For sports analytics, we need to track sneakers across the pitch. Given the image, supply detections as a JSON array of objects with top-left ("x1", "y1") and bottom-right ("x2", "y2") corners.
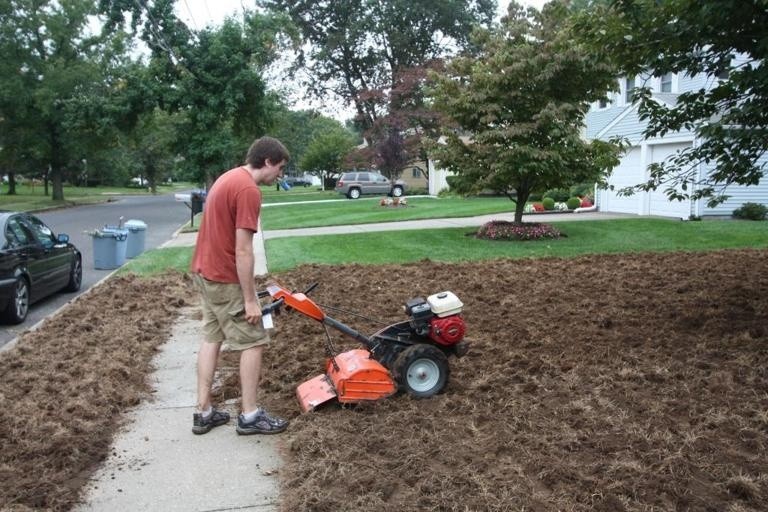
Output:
[
  {"x1": 236, "y1": 408, "x2": 289, "y2": 436},
  {"x1": 192, "y1": 407, "x2": 230, "y2": 435}
]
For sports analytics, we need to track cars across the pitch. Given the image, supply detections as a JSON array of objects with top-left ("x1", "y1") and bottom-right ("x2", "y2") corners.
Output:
[{"x1": 1, "y1": 207, "x2": 84, "y2": 326}]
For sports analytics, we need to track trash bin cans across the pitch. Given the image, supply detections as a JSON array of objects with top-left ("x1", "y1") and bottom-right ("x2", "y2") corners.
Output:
[
  {"x1": 192, "y1": 192, "x2": 205, "y2": 215},
  {"x1": 88, "y1": 219, "x2": 147, "y2": 270}
]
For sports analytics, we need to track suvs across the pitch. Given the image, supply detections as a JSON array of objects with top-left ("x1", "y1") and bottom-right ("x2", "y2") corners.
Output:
[{"x1": 335, "y1": 170, "x2": 411, "y2": 200}]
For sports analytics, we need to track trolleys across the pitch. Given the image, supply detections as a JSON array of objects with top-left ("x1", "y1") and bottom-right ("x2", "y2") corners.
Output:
[{"x1": 245, "y1": 281, "x2": 470, "y2": 416}]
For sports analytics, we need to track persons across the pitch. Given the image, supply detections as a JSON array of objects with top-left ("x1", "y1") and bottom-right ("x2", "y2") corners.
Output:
[{"x1": 189, "y1": 135, "x2": 290, "y2": 434}]
